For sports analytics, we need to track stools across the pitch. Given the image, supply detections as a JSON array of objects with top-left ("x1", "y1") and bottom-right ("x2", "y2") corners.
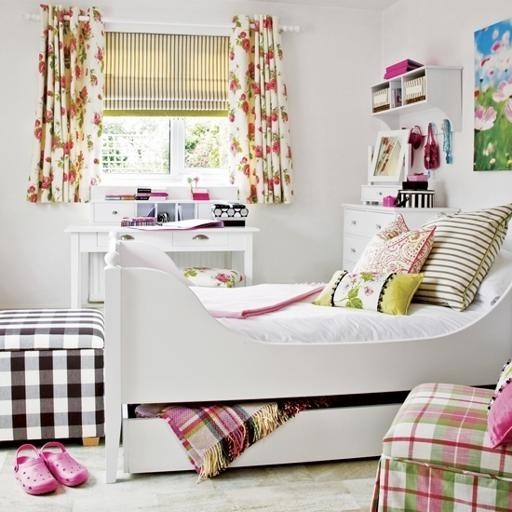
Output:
[
  {"x1": 0, "y1": 307, "x2": 105, "y2": 448},
  {"x1": 179, "y1": 264, "x2": 245, "y2": 290}
]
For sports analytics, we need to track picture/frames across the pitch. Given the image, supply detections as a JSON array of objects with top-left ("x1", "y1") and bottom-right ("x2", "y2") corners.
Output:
[{"x1": 368, "y1": 130, "x2": 412, "y2": 185}]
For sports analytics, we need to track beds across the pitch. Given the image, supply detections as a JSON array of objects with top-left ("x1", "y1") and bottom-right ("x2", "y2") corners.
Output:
[{"x1": 100, "y1": 225, "x2": 511, "y2": 485}]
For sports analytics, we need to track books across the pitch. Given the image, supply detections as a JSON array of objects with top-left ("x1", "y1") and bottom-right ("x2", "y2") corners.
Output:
[{"x1": 119, "y1": 216, "x2": 156, "y2": 225}]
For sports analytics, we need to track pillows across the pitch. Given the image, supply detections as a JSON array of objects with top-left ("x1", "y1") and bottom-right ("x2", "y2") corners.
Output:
[
  {"x1": 416, "y1": 199, "x2": 512, "y2": 309},
  {"x1": 309, "y1": 266, "x2": 423, "y2": 317},
  {"x1": 485, "y1": 356, "x2": 512, "y2": 449},
  {"x1": 475, "y1": 249, "x2": 511, "y2": 305},
  {"x1": 353, "y1": 213, "x2": 440, "y2": 273}
]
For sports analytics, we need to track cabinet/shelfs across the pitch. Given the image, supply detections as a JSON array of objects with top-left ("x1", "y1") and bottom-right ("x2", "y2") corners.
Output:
[
  {"x1": 370, "y1": 66, "x2": 463, "y2": 137},
  {"x1": 360, "y1": 183, "x2": 434, "y2": 206},
  {"x1": 342, "y1": 203, "x2": 456, "y2": 282}
]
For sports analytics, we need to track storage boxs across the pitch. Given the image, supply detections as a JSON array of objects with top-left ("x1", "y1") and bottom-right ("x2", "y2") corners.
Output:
[
  {"x1": 401, "y1": 174, "x2": 429, "y2": 191},
  {"x1": 209, "y1": 202, "x2": 249, "y2": 227}
]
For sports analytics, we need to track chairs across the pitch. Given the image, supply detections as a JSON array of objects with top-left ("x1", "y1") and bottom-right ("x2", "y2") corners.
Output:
[{"x1": 369, "y1": 354, "x2": 512, "y2": 510}]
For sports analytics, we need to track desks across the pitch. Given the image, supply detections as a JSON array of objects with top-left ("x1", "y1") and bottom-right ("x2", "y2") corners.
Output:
[{"x1": 66, "y1": 186, "x2": 261, "y2": 307}]
